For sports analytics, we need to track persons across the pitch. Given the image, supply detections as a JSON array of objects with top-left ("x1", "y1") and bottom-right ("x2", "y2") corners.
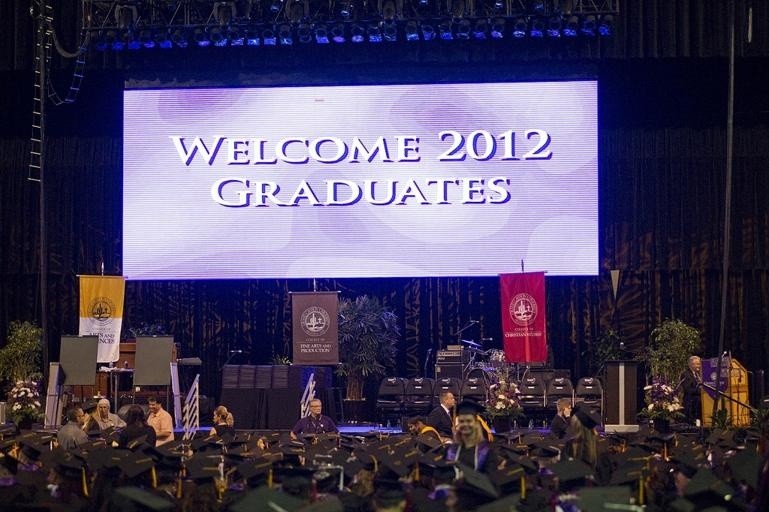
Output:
[{"x1": 0, "y1": 356, "x2": 768, "y2": 512}]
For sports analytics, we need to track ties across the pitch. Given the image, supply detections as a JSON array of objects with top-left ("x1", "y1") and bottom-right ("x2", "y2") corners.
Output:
[{"x1": 694, "y1": 371, "x2": 702, "y2": 385}]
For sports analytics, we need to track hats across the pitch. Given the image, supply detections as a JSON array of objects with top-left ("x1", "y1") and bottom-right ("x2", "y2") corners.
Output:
[{"x1": 0, "y1": 386, "x2": 769, "y2": 511}]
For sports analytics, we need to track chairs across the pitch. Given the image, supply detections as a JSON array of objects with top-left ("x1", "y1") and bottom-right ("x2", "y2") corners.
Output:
[{"x1": 376, "y1": 373, "x2": 604, "y2": 435}]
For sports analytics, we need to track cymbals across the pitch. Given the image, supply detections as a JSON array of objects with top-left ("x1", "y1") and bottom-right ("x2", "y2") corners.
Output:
[
  {"x1": 466, "y1": 347, "x2": 488, "y2": 356},
  {"x1": 461, "y1": 339, "x2": 481, "y2": 347}
]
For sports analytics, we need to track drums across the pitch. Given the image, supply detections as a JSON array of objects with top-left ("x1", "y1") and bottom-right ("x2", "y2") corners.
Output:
[{"x1": 466, "y1": 368, "x2": 490, "y2": 379}]
